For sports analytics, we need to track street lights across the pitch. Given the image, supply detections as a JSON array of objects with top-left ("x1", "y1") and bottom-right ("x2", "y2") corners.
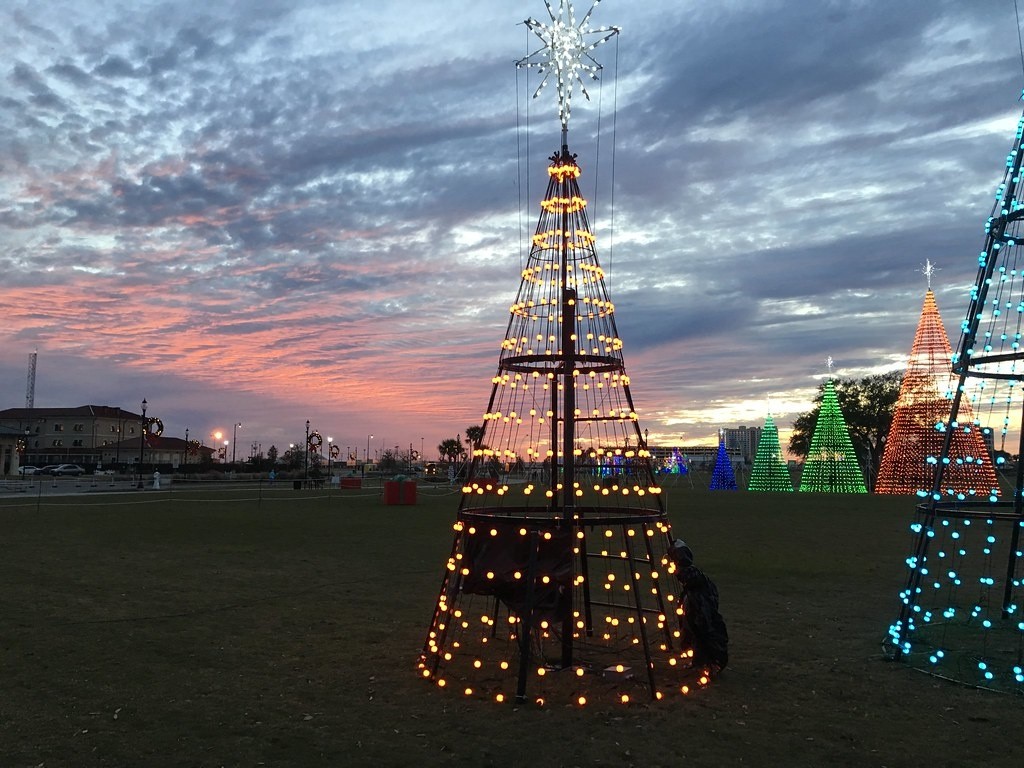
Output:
[
  {"x1": 367, "y1": 433, "x2": 374, "y2": 465},
  {"x1": 452, "y1": 433, "x2": 461, "y2": 478},
  {"x1": 224, "y1": 440, "x2": 229, "y2": 474},
  {"x1": 136, "y1": 397, "x2": 148, "y2": 489},
  {"x1": 326, "y1": 435, "x2": 333, "y2": 480},
  {"x1": 421, "y1": 437, "x2": 424, "y2": 462},
  {"x1": 182, "y1": 428, "x2": 189, "y2": 479},
  {"x1": 231, "y1": 422, "x2": 241, "y2": 474},
  {"x1": 21, "y1": 426, "x2": 31, "y2": 480},
  {"x1": 347, "y1": 446, "x2": 350, "y2": 461},
  {"x1": 303, "y1": 420, "x2": 312, "y2": 487}
]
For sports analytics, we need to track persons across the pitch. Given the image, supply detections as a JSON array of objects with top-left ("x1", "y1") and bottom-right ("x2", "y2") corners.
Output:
[
  {"x1": 152, "y1": 467, "x2": 160, "y2": 489},
  {"x1": 268, "y1": 469, "x2": 277, "y2": 484},
  {"x1": 672, "y1": 538, "x2": 722, "y2": 669}
]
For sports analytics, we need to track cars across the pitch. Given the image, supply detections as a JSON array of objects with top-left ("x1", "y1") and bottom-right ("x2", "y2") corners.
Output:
[
  {"x1": 50, "y1": 464, "x2": 87, "y2": 477},
  {"x1": 33, "y1": 465, "x2": 61, "y2": 475},
  {"x1": 18, "y1": 466, "x2": 39, "y2": 475}
]
